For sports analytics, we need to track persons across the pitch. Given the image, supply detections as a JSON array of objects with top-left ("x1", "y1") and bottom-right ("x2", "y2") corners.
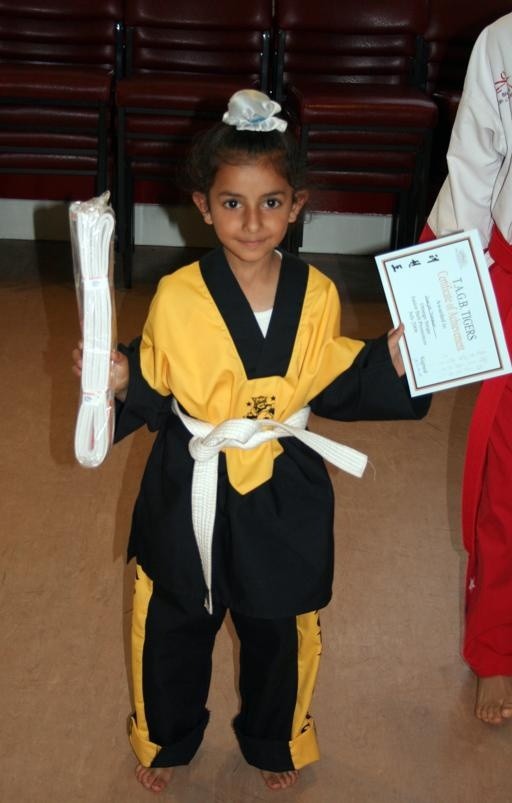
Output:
[
  {"x1": 65, "y1": 86, "x2": 436, "y2": 797},
  {"x1": 412, "y1": 8, "x2": 512, "y2": 731}
]
